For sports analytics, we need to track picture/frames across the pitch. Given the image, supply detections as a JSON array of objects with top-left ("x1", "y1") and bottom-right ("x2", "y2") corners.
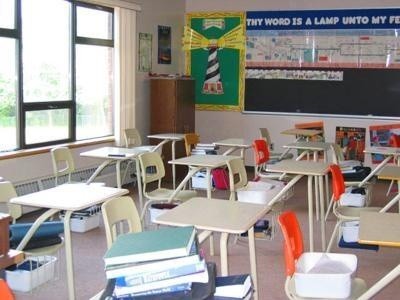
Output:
[{"x1": 185, "y1": 12, "x2": 246, "y2": 112}]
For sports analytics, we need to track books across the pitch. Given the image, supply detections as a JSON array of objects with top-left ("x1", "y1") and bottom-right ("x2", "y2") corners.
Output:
[
  {"x1": 100, "y1": 226, "x2": 256, "y2": 300},
  {"x1": 337, "y1": 156, "x2": 363, "y2": 175},
  {"x1": 62, "y1": 204, "x2": 104, "y2": 219},
  {"x1": 190, "y1": 139, "x2": 219, "y2": 176}
]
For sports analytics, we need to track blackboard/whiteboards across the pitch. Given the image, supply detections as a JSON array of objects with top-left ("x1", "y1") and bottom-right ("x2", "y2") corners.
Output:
[{"x1": 241, "y1": 67, "x2": 400, "y2": 119}]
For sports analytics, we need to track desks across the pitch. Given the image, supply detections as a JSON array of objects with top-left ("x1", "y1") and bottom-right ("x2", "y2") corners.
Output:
[
  {"x1": 0, "y1": 128, "x2": 382, "y2": 300},
  {"x1": 358, "y1": 147, "x2": 400, "y2": 213},
  {"x1": 378, "y1": 166, "x2": 400, "y2": 212},
  {"x1": 356, "y1": 212, "x2": 400, "y2": 300}
]
